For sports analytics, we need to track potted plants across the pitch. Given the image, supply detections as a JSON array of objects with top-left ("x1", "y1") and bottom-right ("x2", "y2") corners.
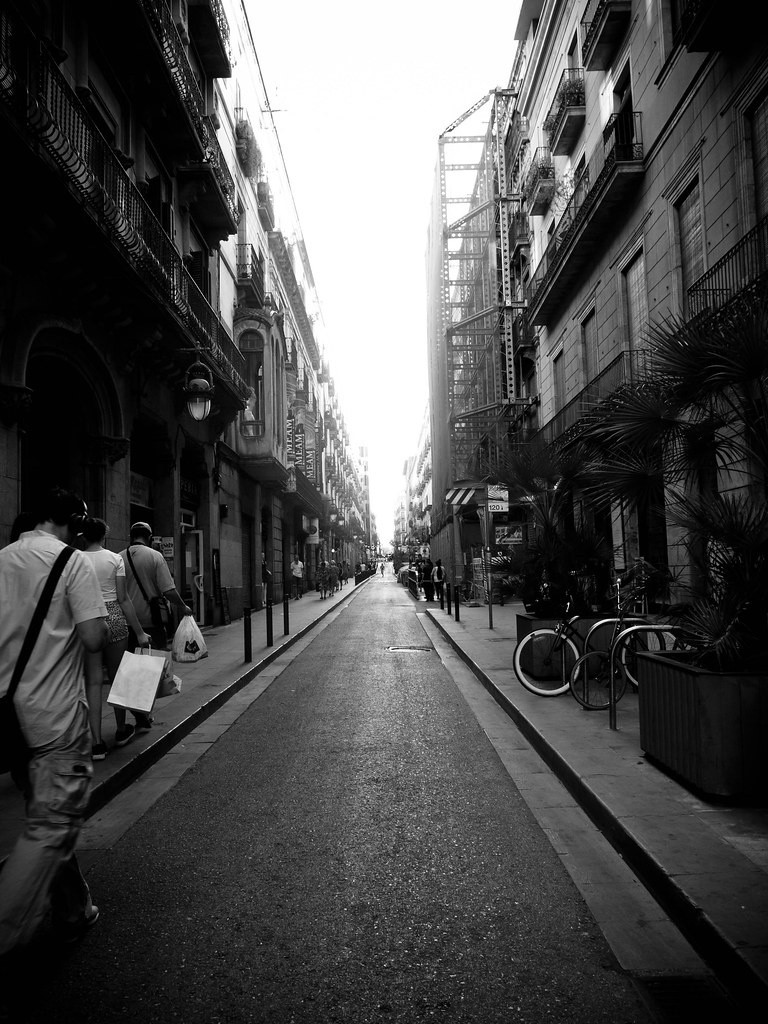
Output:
[
  {"x1": 557, "y1": 261, "x2": 766, "y2": 807},
  {"x1": 477, "y1": 430, "x2": 638, "y2": 679}
]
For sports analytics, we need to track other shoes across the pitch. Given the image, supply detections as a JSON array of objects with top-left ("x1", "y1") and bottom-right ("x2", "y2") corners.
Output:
[
  {"x1": 134, "y1": 720, "x2": 152, "y2": 730},
  {"x1": 58, "y1": 905, "x2": 100, "y2": 936}
]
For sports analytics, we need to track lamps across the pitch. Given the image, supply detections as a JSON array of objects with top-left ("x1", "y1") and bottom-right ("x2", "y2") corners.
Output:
[{"x1": 112, "y1": 343, "x2": 213, "y2": 421}]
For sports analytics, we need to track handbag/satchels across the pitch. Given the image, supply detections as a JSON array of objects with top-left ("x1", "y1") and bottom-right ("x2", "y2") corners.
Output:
[
  {"x1": 0, "y1": 696, "x2": 31, "y2": 774},
  {"x1": 172, "y1": 614, "x2": 209, "y2": 663},
  {"x1": 108, "y1": 641, "x2": 170, "y2": 714},
  {"x1": 134, "y1": 642, "x2": 182, "y2": 699},
  {"x1": 151, "y1": 596, "x2": 171, "y2": 627}
]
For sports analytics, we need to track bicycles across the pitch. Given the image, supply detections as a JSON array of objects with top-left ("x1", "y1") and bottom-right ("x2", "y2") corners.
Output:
[
  {"x1": 569, "y1": 586, "x2": 703, "y2": 711},
  {"x1": 512, "y1": 577, "x2": 671, "y2": 696}
]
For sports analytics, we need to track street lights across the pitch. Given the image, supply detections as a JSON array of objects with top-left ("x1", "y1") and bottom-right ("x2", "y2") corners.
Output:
[{"x1": 168, "y1": 361, "x2": 212, "y2": 630}]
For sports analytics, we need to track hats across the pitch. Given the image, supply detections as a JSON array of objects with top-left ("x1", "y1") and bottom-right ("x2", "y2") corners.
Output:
[{"x1": 130, "y1": 522, "x2": 152, "y2": 536}]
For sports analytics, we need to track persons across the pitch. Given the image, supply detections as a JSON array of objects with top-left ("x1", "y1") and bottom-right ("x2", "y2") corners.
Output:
[
  {"x1": 74, "y1": 517, "x2": 152, "y2": 759},
  {"x1": 0, "y1": 489, "x2": 111, "y2": 960},
  {"x1": 416, "y1": 554, "x2": 445, "y2": 602},
  {"x1": 261, "y1": 552, "x2": 272, "y2": 606},
  {"x1": 290, "y1": 554, "x2": 304, "y2": 600},
  {"x1": 116, "y1": 521, "x2": 193, "y2": 652},
  {"x1": 316, "y1": 558, "x2": 384, "y2": 600}
]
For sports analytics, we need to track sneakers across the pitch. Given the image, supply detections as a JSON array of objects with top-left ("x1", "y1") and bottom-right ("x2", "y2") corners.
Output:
[
  {"x1": 116, "y1": 723, "x2": 135, "y2": 746},
  {"x1": 90, "y1": 738, "x2": 108, "y2": 760}
]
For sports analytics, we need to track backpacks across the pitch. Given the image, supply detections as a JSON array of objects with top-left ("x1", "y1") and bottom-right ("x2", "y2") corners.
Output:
[{"x1": 437, "y1": 567, "x2": 442, "y2": 581}]
